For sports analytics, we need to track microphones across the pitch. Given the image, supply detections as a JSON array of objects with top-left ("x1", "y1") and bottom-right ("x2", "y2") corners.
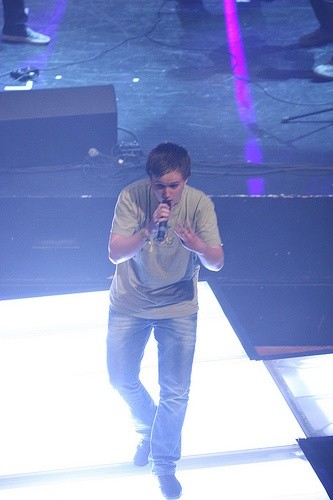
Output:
[{"x1": 157, "y1": 200, "x2": 171, "y2": 241}]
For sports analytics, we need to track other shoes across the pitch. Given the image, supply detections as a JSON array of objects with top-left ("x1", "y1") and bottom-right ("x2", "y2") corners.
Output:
[
  {"x1": 300, "y1": 27, "x2": 333, "y2": 46},
  {"x1": 313, "y1": 65, "x2": 333, "y2": 78}
]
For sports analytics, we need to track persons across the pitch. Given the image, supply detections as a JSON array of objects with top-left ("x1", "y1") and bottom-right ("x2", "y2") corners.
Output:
[
  {"x1": 105, "y1": 143, "x2": 226, "y2": 500},
  {"x1": 0, "y1": 0, "x2": 333, "y2": 80}
]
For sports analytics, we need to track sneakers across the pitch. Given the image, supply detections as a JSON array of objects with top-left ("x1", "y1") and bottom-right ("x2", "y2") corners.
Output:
[
  {"x1": 133, "y1": 430, "x2": 150, "y2": 467},
  {"x1": 157, "y1": 471, "x2": 183, "y2": 500},
  {"x1": 2, "y1": 28, "x2": 50, "y2": 43}
]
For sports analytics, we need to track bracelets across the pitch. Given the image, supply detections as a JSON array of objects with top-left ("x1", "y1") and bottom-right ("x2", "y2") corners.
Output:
[{"x1": 140, "y1": 230, "x2": 148, "y2": 243}]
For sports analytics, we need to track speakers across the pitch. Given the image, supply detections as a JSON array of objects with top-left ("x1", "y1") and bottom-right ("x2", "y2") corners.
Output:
[{"x1": 0, "y1": 84, "x2": 117, "y2": 173}]
